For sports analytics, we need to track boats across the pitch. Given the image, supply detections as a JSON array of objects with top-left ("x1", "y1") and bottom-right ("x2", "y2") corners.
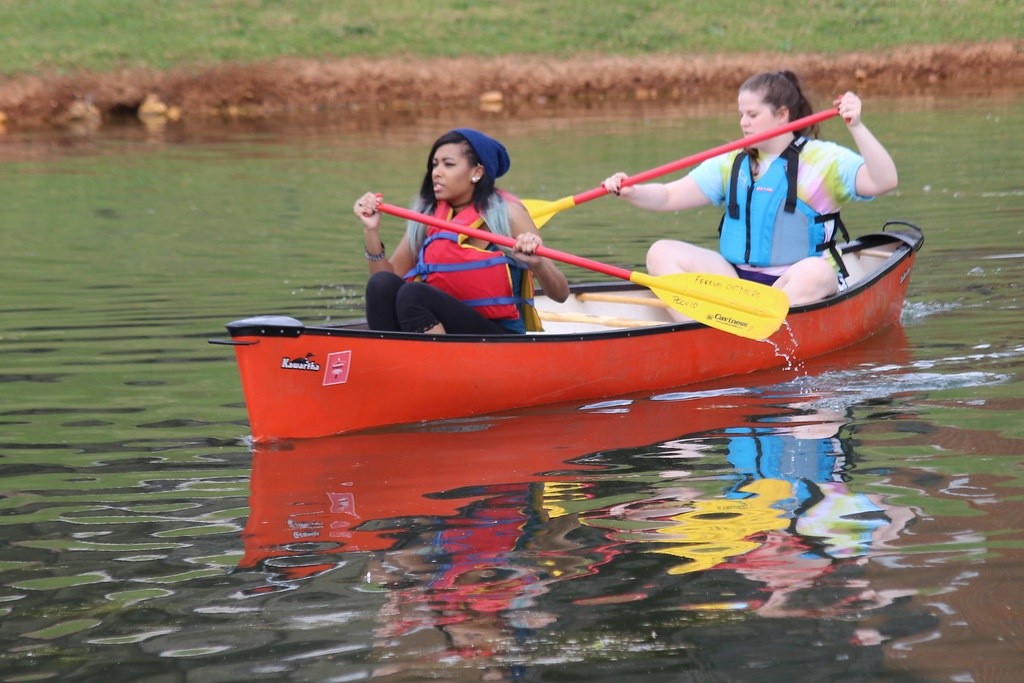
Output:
[
  {"x1": 208, "y1": 222, "x2": 924, "y2": 441},
  {"x1": 230, "y1": 323, "x2": 916, "y2": 576}
]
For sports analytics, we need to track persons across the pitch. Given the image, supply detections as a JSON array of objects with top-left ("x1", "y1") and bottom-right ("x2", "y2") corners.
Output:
[
  {"x1": 354, "y1": 130, "x2": 570, "y2": 335},
  {"x1": 600, "y1": 70, "x2": 897, "y2": 323}
]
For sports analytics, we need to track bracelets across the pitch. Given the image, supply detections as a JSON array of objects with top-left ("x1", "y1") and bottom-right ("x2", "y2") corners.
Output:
[{"x1": 364, "y1": 241, "x2": 385, "y2": 261}]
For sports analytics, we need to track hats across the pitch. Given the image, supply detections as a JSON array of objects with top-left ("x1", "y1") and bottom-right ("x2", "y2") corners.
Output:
[{"x1": 450, "y1": 128, "x2": 510, "y2": 184}]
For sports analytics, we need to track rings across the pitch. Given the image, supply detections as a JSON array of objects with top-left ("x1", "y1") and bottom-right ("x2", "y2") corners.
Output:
[
  {"x1": 359, "y1": 202, "x2": 363, "y2": 206},
  {"x1": 529, "y1": 237, "x2": 534, "y2": 240}
]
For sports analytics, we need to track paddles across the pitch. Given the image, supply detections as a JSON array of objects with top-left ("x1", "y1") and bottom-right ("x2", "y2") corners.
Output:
[
  {"x1": 519, "y1": 93, "x2": 854, "y2": 230},
  {"x1": 362, "y1": 190, "x2": 792, "y2": 341}
]
